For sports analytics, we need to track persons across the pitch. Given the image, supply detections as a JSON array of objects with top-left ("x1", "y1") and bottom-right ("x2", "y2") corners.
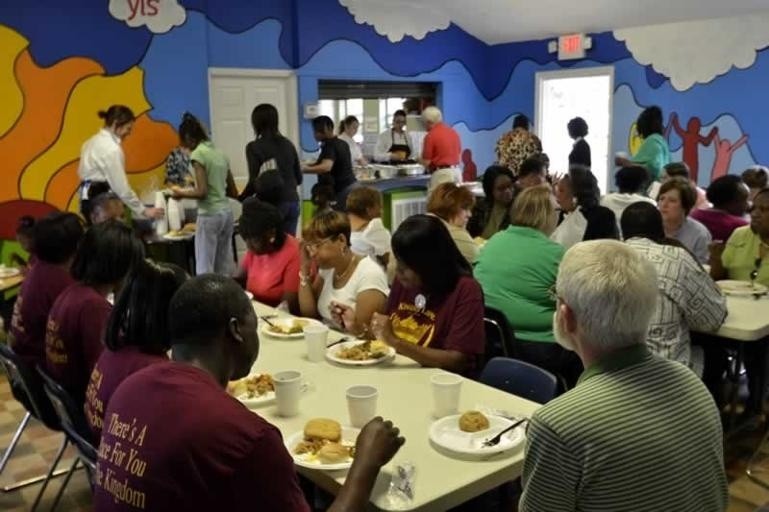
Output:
[
  {"x1": 74, "y1": 105, "x2": 166, "y2": 228},
  {"x1": 231, "y1": 198, "x2": 316, "y2": 319},
  {"x1": 297, "y1": 209, "x2": 390, "y2": 337},
  {"x1": 166, "y1": 124, "x2": 213, "y2": 201},
  {"x1": 494, "y1": 114, "x2": 543, "y2": 177},
  {"x1": 335, "y1": 114, "x2": 367, "y2": 167},
  {"x1": 705, "y1": 185, "x2": 769, "y2": 288},
  {"x1": 330, "y1": 215, "x2": 486, "y2": 373},
  {"x1": 425, "y1": 181, "x2": 480, "y2": 271},
  {"x1": 742, "y1": 164, "x2": 769, "y2": 195},
  {"x1": 418, "y1": 107, "x2": 464, "y2": 170},
  {"x1": 166, "y1": 112, "x2": 239, "y2": 280},
  {"x1": 9, "y1": 213, "x2": 87, "y2": 374},
  {"x1": 97, "y1": 272, "x2": 407, "y2": 511},
  {"x1": 7, "y1": 216, "x2": 38, "y2": 268},
  {"x1": 516, "y1": 156, "x2": 547, "y2": 188},
  {"x1": 599, "y1": 167, "x2": 660, "y2": 235},
  {"x1": 518, "y1": 238, "x2": 733, "y2": 511},
  {"x1": 704, "y1": 174, "x2": 751, "y2": 218},
  {"x1": 474, "y1": 183, "x2": 585, "y2": 385},
  {"x1": 533, "y1": 152, "x2": 552, "y2": 183},
  {"x1": 46, "y1": 218, "x2": 146, "y2": 413},
  {"x1": 86, "y1": 261, "x2": 190, "y2": 439},
  {"x1": 618, "y1": 201, "x2": 727, "y2": 368},
  {"x1": 87, "y1": 193, "x2": 127, "y2": 228},
  {"x1": 467, "y1": 164, "x2": 524, "y2": 241},
  {"x1": 565, "y1": 117, "x2": 592, "y2": 173},
  {"x1": 305, "y1": 115, "x2": 358, "y2": 204},
  {"x1": 552, "y1": 166, "x2": 622, "y2": 242},
  {"x1": 239, "y1": 104, "x2": 304, "y2": 239},
  {"x1": 373, "y1": 109, "x2": 418, "y2": 164},
  {"x1": 663, "y1": 162, "x2": 712, "y2": 213},
  {"x1": 614, "y1": 104, "x2": 670, "y2": 180},
  {"x1": 655, "y1": 177, "x2": 713, "y2": 267}
]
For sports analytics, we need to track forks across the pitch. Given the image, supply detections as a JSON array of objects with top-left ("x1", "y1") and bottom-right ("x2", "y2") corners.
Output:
[{"x1": 482, "y1": 418, "x2": 528, "y2": 447}]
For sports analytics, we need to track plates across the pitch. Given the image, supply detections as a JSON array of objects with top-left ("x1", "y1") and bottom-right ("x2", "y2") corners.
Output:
[
  {"x1": 282, "y1": 425, "x2": 364, "y2": 470},
  {"x1": 428, "y1": 413, "x2": 527, "y2": 456},
  {"x1": 163, "y1": 233, "x2": 193, "y2": 241},
  {"x1": 263, "y1": 317, "x2": 328, "y2": 338},
  {"x1": 327, "y1": 340, "x2": 396, "y2": 365},
  {"x1": 0, "y1": 267, "x2": 21, "y2": 278},
  {"x1": 715, "y1": 280, "x2": 767, "y2": 296},
  {"x1": 225, "y1": 373, "x2": 276, "y2": 405}
]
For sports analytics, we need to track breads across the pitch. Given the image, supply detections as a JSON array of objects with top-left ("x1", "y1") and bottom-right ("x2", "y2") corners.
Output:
[
  {"x1": 305, "y1": 418, "x2": 342, "y2": 445},
  {"x1": 459, "y1": 411, "x2": 488, "y2": 432},
  {"x1": 318, "y1": 444, "x2": 347, "y2": 465}
]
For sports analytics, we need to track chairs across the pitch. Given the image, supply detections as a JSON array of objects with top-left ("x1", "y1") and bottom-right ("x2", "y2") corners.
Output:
[
  {"x1": 34, "y1": 365, "x2": 81, "y2": 512},
  {"x1": 51, "y1": 420, "x2": 98, "y2": 512},
  {"x1": 0, "y1": 344, "x2": 67, "y2": 512},
  {"x1": 480, "y1": 357, "x2": 556, "y2": 405},
  {"x1": 482, "y1": 305, "x2": 515, "y2": 357}
]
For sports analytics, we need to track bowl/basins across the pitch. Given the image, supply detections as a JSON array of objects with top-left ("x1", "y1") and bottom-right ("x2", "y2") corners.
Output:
[
  {"x1": 399, "y1": 163, "x2": 426, "y2": 177},
  {"x1": 372, "y1": 164, "x2": 398, "y2": 180},
  {"x1": 353, "y1": 166, "x2": 373, "y2": 181}
]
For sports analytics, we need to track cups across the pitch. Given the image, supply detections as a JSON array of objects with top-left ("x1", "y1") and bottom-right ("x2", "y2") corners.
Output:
[
  {"x1": 153, "y1": 192, "x2": 168, "y2": 236},
  {"x1": 168, "y1": 196, "x2": 187, "y2": 231},
  {"x1": 429, "y1": 373, "x2": 463, "y2": 418},
  {"x1": 273, "y1": 369, "x2": 305, "y2": 417},
  {"x1": 346, "y1": 385, "x2": 380, "y2": 427},
  {"x1": 303, "y1": 325, "x2": 329, "y2": 361}
]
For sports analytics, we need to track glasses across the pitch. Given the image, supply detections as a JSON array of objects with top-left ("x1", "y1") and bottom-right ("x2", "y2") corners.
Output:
[
  {"x1": 495, "y1": 182, "x2": 513, "y2": 191},
  {"x1": 548, "y1": 286, "x2": 565, "y2": 306},
  {"x1": 750, "y1": 257, "x2": 761, "y2": 280},
  {"x1": 304, "y1": 235, "x2": 335, "y2": 251}
]
